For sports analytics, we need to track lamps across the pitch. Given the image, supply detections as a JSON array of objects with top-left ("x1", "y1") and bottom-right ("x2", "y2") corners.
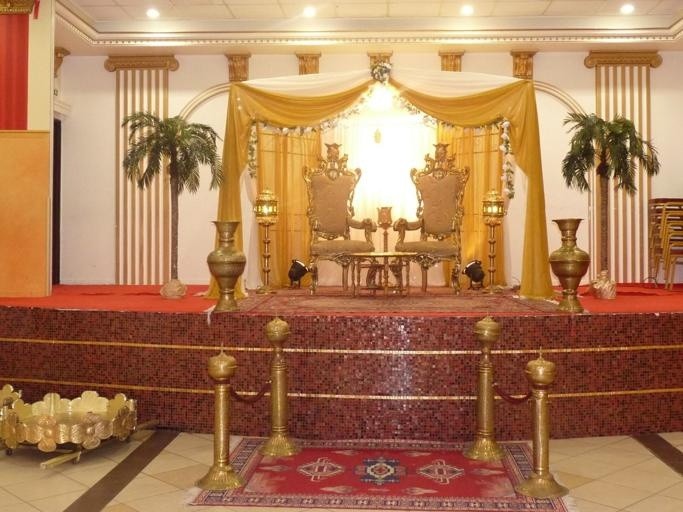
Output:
[
  {"x1": 481, "y1": 187, "x2": 505, "y2": 293},
  {"x1": 252, "y1": 182, "x2": 278, "y2": 295}
]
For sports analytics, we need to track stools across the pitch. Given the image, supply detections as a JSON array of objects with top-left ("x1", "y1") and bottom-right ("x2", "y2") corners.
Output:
[{"x1": 645, "y1": 198, "x2": 683, "y2": 290}]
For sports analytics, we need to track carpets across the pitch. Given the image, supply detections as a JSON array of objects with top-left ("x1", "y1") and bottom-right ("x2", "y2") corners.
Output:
[
  {"x1": 202, "y1": 287, "x2": 589, "y2": 315},
  {"x1": 181, "y1": 436, "x2": 576, "y2": 511}
]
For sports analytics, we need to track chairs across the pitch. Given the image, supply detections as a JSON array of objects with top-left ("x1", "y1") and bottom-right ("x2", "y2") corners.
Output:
[{"x1": 301, "y1": 142, "x2": 470, "y2": 298}]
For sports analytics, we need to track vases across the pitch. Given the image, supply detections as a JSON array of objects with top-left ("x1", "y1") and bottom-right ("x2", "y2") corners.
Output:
[
  {"x1": 548, "y1": 218, "x2": 590, "y2": 313},
  {"x1": 206, "y1": 220, "x2": 245, "y2": 312}
]
